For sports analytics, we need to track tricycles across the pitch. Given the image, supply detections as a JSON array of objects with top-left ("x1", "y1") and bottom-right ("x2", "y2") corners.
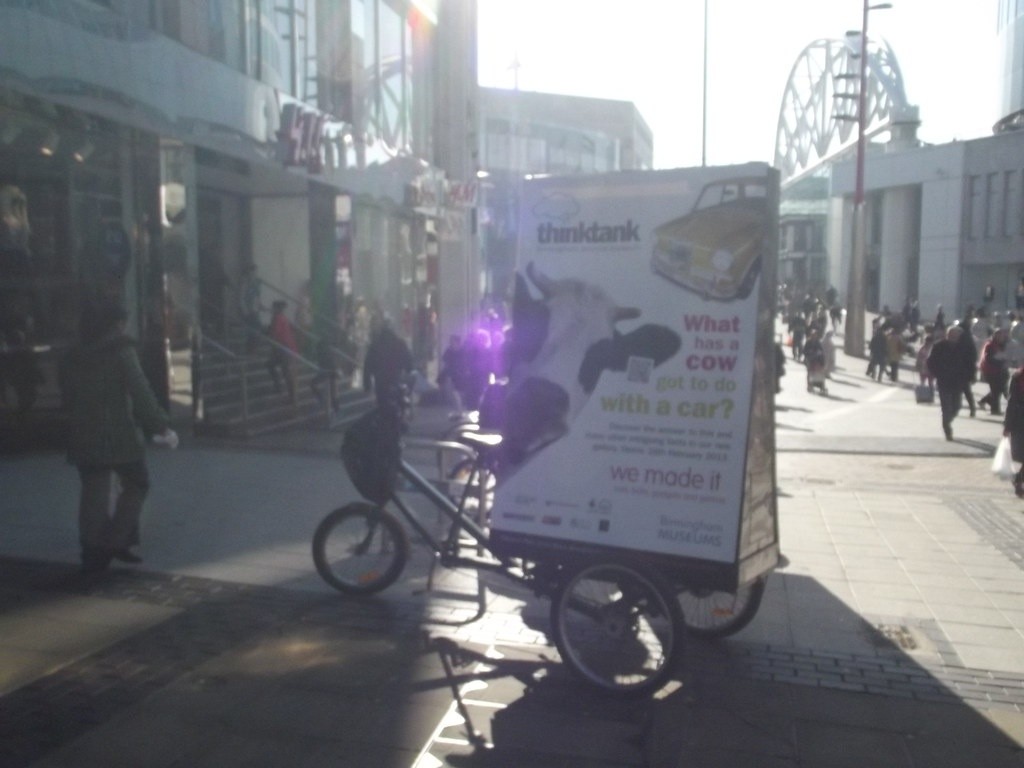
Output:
[{"x1": 307, "y1": 353, "x2": 790, "y2": 696}]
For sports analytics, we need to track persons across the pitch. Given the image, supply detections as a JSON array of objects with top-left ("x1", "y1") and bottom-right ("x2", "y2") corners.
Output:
[
  {"x1": 346, "y1": 301, "x2": 511, "y2": 428},
  {"x1": 774, "y1": 274, "x2": 1024, "y2": 501},
  {"x1": 0, "y1": 150, "x2": 136, "y2": 411},
  {"x1": 265, "y1": 297, "x2": 301, "y2": 405},
  {"x1": 242, "y1": 278, "x2": 270, "y2": 351},
  {"x1": 310, "y1": 332, "x2": 341, "y2": 416},
  {"x1": 64, "y1": 298, "x2": 177, "y2": 572}
]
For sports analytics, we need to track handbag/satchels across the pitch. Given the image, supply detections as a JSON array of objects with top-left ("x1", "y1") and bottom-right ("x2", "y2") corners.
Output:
[
  {"x1": 992, "y1": 436, "x2": 1016, "y2": 481},
  {"x1": 912, "y1": 365, "x2": 933, "y2": 403}
]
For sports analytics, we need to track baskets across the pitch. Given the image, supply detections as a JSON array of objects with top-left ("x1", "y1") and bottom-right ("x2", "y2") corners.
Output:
[{"x1": 342, "y1": 412, "x2": 399, "y2": 503}]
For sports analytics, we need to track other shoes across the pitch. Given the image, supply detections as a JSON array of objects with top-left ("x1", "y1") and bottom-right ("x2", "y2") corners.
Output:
[{"x1": 101, "y1": 540, "x2": 143, "y2": 564}]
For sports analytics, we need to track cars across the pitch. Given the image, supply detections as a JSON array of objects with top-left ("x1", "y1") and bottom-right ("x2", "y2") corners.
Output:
[{"x1": 650, "y1": 169, "x2": 771, "y2": 303}]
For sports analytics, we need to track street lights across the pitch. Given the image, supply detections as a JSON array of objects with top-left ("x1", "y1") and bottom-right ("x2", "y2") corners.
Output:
[{"x1": 831, "y1": 0, "x2": 894, "y2": 356}]
